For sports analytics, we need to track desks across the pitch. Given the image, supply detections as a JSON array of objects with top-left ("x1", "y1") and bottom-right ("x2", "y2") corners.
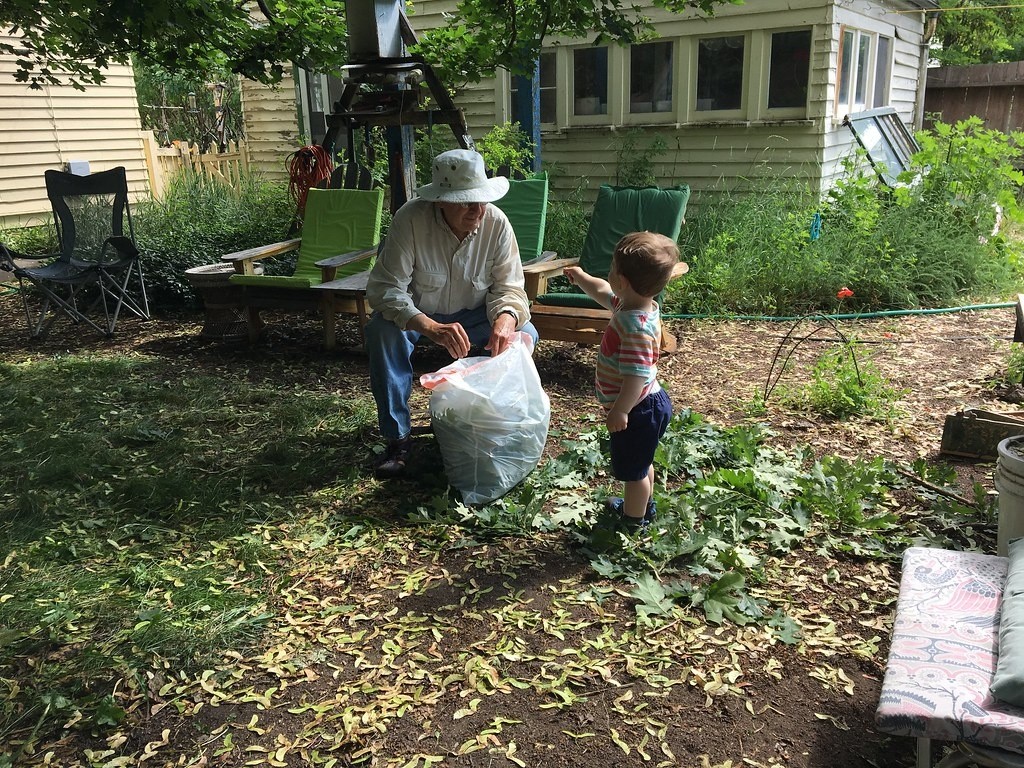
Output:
[{"x1": 310, "y1": 270, "x2": 371, "y2": 344}]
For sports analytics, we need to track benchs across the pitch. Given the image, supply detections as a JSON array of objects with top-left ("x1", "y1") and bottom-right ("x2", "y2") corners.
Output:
[{"x1": 876, "y1": 538, "x2": 1024, "y2": 768}]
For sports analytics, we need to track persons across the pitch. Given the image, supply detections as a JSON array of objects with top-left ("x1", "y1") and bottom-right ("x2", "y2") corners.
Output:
[
  {"x1": 562, "y1": 228, "x2": 680, "y2": 540},
  {"x1": 366, "y1": 148, "x2": 540, "y2": 479}
]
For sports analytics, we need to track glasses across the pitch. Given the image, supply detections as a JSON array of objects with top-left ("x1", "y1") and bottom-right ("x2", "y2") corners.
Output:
[{"x1": 459, "y1": 203, "x2": 472, "y2": 208}]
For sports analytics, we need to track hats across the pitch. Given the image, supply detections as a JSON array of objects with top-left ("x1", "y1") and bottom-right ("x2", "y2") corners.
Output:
[{"x1": 413, "y1": 149, "x2": 512, "y2": 204}]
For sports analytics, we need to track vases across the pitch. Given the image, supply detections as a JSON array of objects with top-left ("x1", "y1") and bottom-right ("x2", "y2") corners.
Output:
[
  {"x1": 996, "y1": 436, "x2": 1024, "y2": 555},
  {"x1": 185, "y1": 263, "x2": 265, "y2": 308}
]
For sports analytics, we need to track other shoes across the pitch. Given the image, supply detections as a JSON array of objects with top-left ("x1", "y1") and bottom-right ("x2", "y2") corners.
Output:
[{"x1": 375, "y1": 434, "x2": 410, "y2": 477}]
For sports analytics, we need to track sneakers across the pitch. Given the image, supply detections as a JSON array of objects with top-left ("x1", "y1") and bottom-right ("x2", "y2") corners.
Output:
[
  {"x1": 599, "y1": 514, "x2": 650, "y2": 537},
  {"x1": 606, "y1": 495, "x2": 659, "y2": 522}
]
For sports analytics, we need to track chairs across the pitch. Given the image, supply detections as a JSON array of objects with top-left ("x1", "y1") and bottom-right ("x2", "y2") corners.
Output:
[
  {"x1": 490, "y1": 179, "x2": 557, "y2": 268},
  {"x1": 522, "y1": 183, "x2": 690, "y2": 353},
  {"x1": 1, "y1": 167, "x2": 151, "y2": 342},
  {"x1": 221, "y1": 186, "x2": 385, "y2": 351}
]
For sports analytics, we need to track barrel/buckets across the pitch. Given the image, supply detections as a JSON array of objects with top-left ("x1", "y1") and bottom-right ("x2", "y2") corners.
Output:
[{"x1": 994, "y1": 435, "x2": 1024, "y2": 557}]
